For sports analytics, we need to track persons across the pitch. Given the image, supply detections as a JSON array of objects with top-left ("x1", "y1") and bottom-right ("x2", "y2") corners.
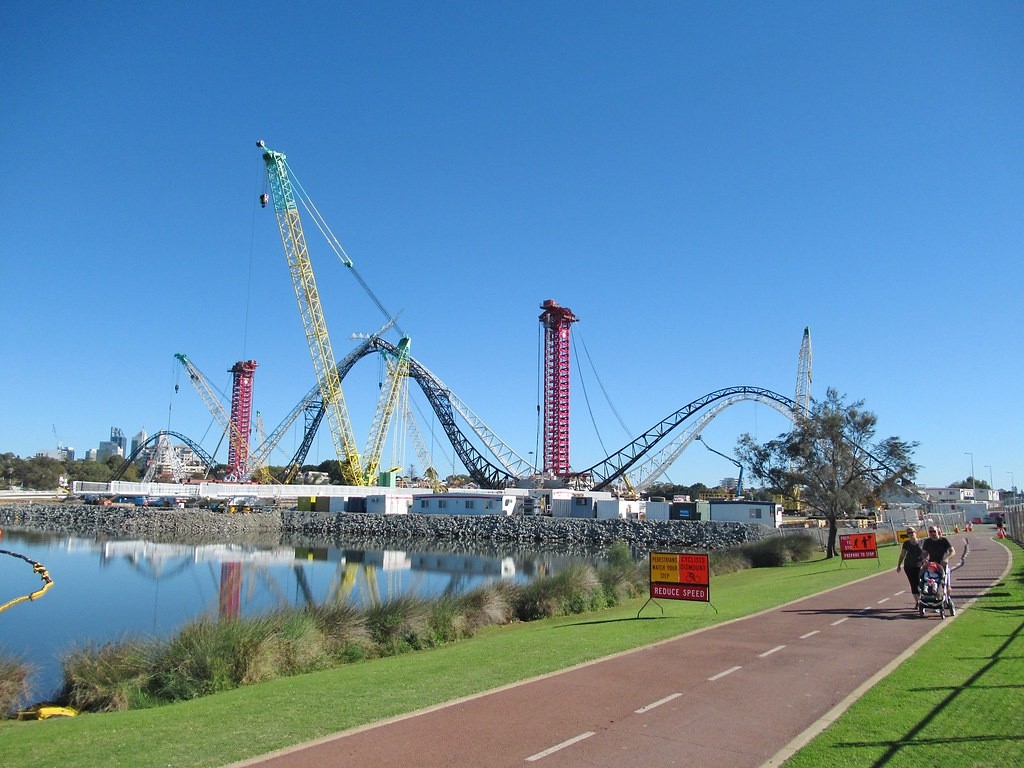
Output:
[
  {"x1": 995, "y1": 513, "x2": 1004, "y2": 530},
  {"x1": 896, "y1": 525, "x2": 956, "y2": 612}
]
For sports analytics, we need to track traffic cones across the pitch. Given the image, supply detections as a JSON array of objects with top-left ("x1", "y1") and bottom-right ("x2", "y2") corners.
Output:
[
  {"x1": 965, "y1": 520, "x2": 973, "y2": 532},
  {"x1": 937, "y1": 526, "x2": 942, "y2": 537},
  {"x1": 955, "y1": 524, "x2": 959, "y2": 533},
  {"x1": 999, "y1": 528, "x2": 1007, "y2": 539}
]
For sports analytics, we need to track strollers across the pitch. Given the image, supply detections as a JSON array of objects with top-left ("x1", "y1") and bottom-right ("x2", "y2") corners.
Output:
[{"x1": 916, "y1": 558, "x2": 956, "y2": 620}]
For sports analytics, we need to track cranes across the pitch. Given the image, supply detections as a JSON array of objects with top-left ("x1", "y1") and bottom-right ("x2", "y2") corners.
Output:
[
  {"x1": 169, "y1": 352, "x2": 302, "y2": 484},
  {"x1": 781, "y1": 326, "x2": 812, "y2": 516},
  {"x1": 379, "y1": 348, "x2": 449, "y2": 496},
  {"x1": 256, "y1": 141, "x2": 413, "y2": 486},
  {"x1": 245, "y1": 554, "x2": 384, "y2": 614}
]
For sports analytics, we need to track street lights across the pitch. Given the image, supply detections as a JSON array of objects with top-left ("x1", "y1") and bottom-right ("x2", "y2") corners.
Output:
[
  {"x1": 529, "y1": 451, "x2": 534, "y2": 475},
  {"x1": 984, "y1": 466, "x2": 994, "y2": 510},
  {"x1": 964, "y1": 453, "x2": 976, "y2": 503},
  {"x1": 1007, "y1": 472, "x2": 1015, "y2": 512}
]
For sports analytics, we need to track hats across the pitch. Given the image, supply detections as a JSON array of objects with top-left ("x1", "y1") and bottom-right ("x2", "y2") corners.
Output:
[{"x1": 925, "y1": 562, "x2": 939, "y2": 571}]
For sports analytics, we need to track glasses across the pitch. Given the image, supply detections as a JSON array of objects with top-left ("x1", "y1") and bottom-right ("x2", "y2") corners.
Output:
[
  {"x1": 929, "y1": 530, "x2": 936, "y2": 533},
  {"x1": 907, "y1": 532, "x2": 914, "y2": 534}
]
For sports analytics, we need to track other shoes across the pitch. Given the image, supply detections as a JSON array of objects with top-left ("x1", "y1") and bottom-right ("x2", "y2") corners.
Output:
[
  {"x1": 915, "y1": 604, "x2": 919, "y2": 609},
  {"x1": 923, "y1": 590, "x2": 928, "y2": 594},
  {"x1": 934, "y1": 609, "x2": 939, "y2": 613},
  {"x1": 932, "y1": 589, "x2": 937, "y2": 594}
]
